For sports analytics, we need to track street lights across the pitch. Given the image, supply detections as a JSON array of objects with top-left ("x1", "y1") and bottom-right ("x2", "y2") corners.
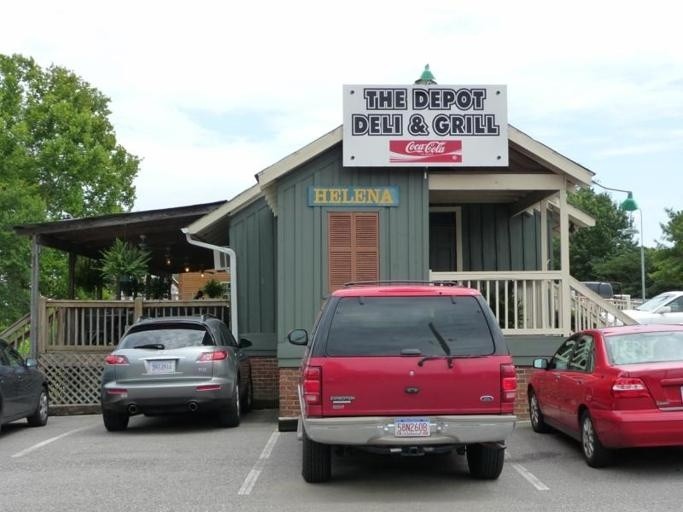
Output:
[{"x1": 630, "y1": 207, "x2": 645, "y2": 298}]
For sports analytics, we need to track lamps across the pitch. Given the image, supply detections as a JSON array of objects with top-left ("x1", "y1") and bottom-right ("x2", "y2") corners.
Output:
[
  {"x1": 591, "y1": 179, "x2": 638, "y2": 211},
  {"x1": 414, "y1": 63, "x2": 437, "y2": 86}
]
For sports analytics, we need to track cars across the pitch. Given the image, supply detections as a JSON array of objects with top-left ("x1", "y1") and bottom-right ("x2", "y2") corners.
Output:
[
  {"x1": 527, "y1": 282, "x2": 682, "y2": 469},
  {"x1": 0, "y1": 338, "x2": 49, "y2": 427},
  {"x1": 101, "y1": 313, "x2": 252, "y2": 432},
  {"x1": 287, "y1": 280, "x2": 518, "y2": 483}
]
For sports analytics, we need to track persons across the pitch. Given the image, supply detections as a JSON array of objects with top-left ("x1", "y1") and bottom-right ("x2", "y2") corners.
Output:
[{"x1": 192, "y1": 291, "x2": 206, "y2": 315}]
